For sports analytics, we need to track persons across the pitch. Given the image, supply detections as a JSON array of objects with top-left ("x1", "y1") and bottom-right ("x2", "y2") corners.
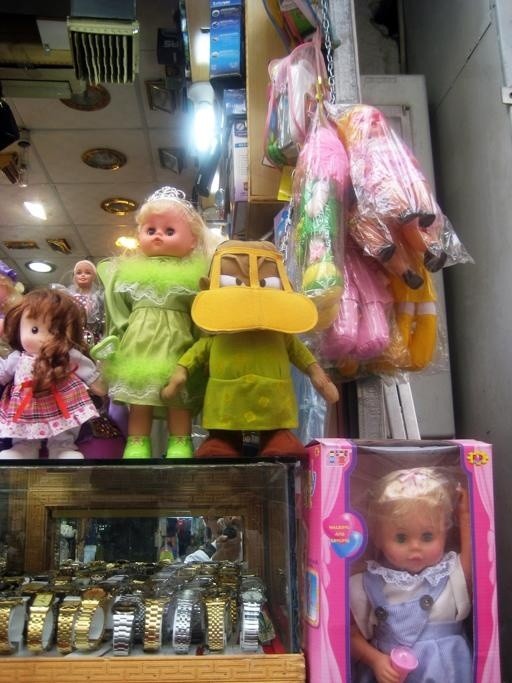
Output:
[
  {"x1": 275, "y1": 122, "x2": 393, "y2": 378},
  {"x1": 60, "y1": 255, "x2": 108, "y2": 350},
  {"x1": 0, "y1": 285, "x2": 104, "y2": 469},
  {"x1": 339, "y1": 463, "x2": 480, "y2": 681},
  {"x1": 337, "y1": 105, "x2": 448, "y2": 290},
  {"x1": 95, "y1": 183, "x2": 214, "y2": 467},
  {"x1": 180, "y1": 516, "x2": 242, "y2": 563}
]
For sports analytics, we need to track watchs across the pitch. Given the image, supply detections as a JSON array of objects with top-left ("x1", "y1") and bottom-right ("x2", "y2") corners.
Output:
[{"x1": 0, "y1": 557, "x2": 268, "y2": 655}]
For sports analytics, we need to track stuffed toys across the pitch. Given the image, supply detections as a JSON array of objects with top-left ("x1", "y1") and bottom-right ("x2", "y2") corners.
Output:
[
  {"x1": 263, "y1": 31, "x2": 453, "y2": 389},
  {"x1": 0, "y1": 259, "x2": 26, "y2": 363},
  {"x1": 160, "y1": 235, "x2": 344, "y2": 467}
]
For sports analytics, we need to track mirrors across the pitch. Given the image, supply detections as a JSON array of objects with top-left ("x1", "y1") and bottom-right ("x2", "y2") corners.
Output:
[{"x1": 48, "y1": 507, "x2": 244, "y2": 568}]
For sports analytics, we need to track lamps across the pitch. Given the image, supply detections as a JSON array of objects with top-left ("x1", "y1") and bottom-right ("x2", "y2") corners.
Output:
[
  {"x1": 146, "y1": 81, "x2": 176, "y2": 114},
  {"x1": 158, "y1": 148, "x2": 186, "y2": 175}
]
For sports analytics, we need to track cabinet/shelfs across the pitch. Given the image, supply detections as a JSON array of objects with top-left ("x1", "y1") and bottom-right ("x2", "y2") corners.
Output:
[
  {"x1": 248, "y1": 0, "x2": 302, "y2": 243},
  {"x1": 0, "y1": 459, "x2": 309, "y2": 683}
]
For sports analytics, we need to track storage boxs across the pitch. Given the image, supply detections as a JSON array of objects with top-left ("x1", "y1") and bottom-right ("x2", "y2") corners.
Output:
[{"x1": 208, "y1": 0, "x2": 248, "y2": 240}]
